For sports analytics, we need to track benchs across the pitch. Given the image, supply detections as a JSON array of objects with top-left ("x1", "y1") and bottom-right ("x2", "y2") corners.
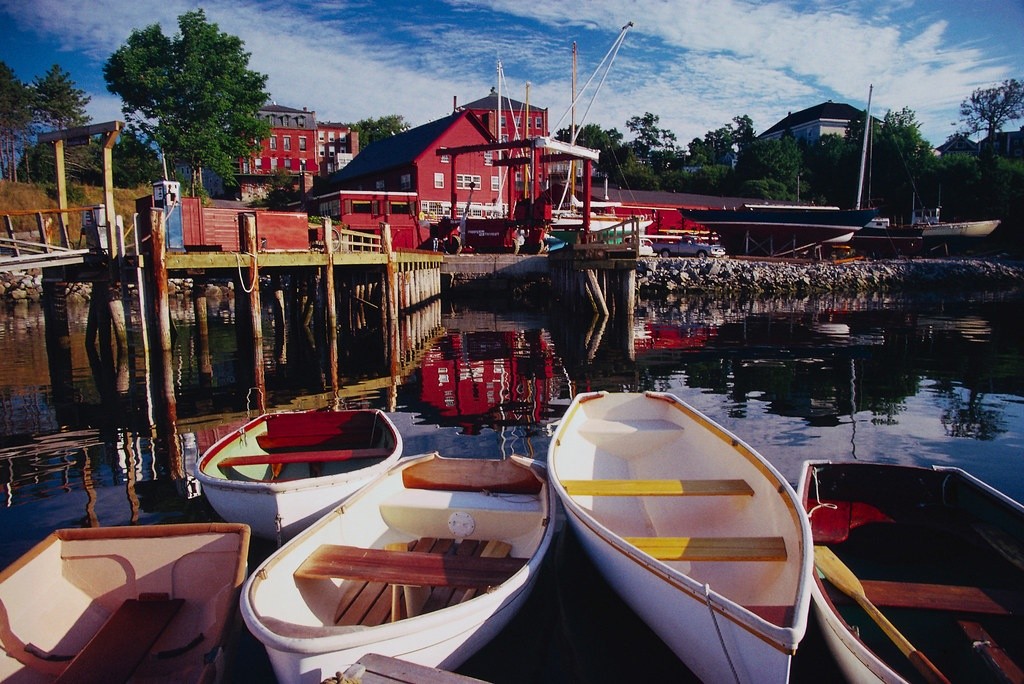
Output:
[
  {"x1": 295, "y1": 544, "x2": 529, "y2": 621},
  {"x1": 218, "y1": 448, "x2": 392, "y2": 478},
  {"x1": 46, "y1": 598, "x2": 187, "y2": 684},
  {"x1": 824, "y1": 578, "x2": 1024, "y2": 617},
  {"x1": 623, "y1": 537, "x2": 787, "y2": 563},
  {"x1": 258, "y1": 429, "x2": 382, "y2": 449},
  {"x1": 561, "y1": 480, "x2": 755, "y2": 497}
]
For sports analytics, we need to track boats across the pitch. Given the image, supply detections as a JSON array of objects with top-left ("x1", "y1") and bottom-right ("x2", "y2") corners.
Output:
[
  {"x1": 0, "y1": 391, "x2": 1024, "y2": 684},
  {"x1": 679, "y1": 84, "x2": 1001, "y2": 264}
]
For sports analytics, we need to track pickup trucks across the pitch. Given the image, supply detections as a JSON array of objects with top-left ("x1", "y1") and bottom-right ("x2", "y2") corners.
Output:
[{"x1": 652, "y1": 236, "x2": 726, "y2": 258}]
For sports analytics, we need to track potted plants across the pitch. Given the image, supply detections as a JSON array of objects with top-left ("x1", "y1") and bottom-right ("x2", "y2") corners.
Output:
[{"x1": 308, "y1": 215, "x2": 343, "y2": 253}]
